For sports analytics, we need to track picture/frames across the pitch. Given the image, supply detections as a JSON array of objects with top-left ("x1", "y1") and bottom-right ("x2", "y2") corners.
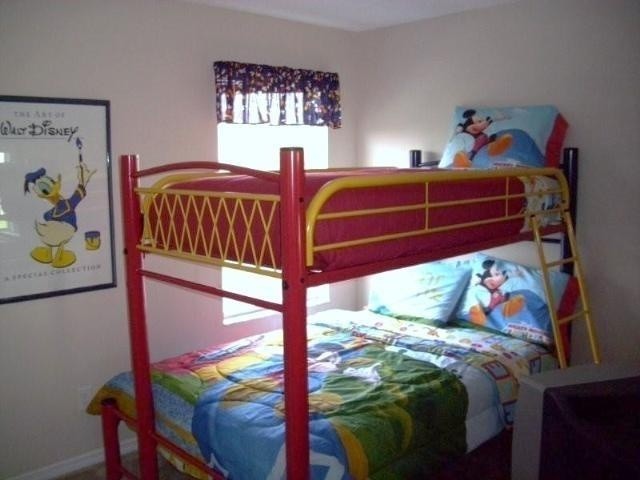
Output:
[{"x1": 0, "y1": 95, "x2": 117, "y2": 305}]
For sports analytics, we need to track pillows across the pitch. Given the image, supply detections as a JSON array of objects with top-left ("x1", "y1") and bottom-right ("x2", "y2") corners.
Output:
[
  {"x1": 436, "y1": 106, "x2": 564, "y2": 173},
  {"x1": 366, "y1": 253, "x2": 572, "y2": 347}
]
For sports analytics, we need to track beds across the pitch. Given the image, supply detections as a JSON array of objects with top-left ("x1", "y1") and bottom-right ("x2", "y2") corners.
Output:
[{"x1": 97, "y1": 146, "x2": 605, "y2": 480}]
